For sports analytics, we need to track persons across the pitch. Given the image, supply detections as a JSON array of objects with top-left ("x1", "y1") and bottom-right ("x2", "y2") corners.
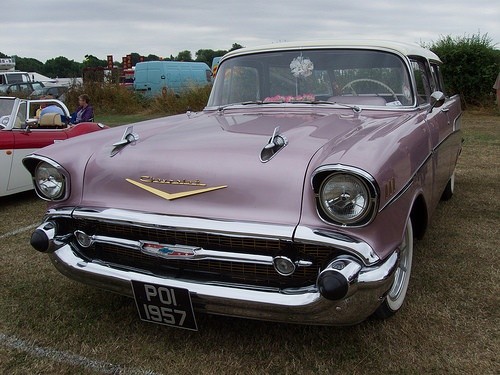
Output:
[
  {"x1": 74, "y1": 94, "x2": 94, "y2": 122},
  {"x1": 32, "y1": 95, "x2": 65, "y2": 118}
]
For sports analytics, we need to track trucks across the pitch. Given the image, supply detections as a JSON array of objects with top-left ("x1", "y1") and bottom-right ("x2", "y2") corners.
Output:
[{"x1": 0, "y1": 73, "x2": 35, "y2": 95}]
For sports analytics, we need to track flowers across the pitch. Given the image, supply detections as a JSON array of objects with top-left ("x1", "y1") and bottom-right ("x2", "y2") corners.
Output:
[
  {"x1": 290, "y1": 56, "x2": 313, "y2": 77},
  {"x1": 265, "y1": 93, "x2": 316, "y2": 101}
]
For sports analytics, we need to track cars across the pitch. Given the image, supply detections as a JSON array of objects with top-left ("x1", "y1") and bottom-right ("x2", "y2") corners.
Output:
[
  {"x1": 4, "y1": 81, "x2": 45, "y2": 97},
  {"x1": 27, "y1": 86, "x2": 68, "y2": 99},
  {"x1": 22, "y1": 40, "x2": 464, "y2": 327},
  {"x1": 0, "y1": 97, "x2": 111, "y2": 194}
]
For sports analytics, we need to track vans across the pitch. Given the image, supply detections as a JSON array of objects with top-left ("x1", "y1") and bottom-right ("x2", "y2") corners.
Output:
[{"x1": 134, "y1": 62, "x2": 214, "y2": 97}]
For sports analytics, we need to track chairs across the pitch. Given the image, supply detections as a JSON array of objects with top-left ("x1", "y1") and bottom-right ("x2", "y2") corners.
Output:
[{"x1": 40, "y1": 112, "x2": 61, "y2": 129}]
